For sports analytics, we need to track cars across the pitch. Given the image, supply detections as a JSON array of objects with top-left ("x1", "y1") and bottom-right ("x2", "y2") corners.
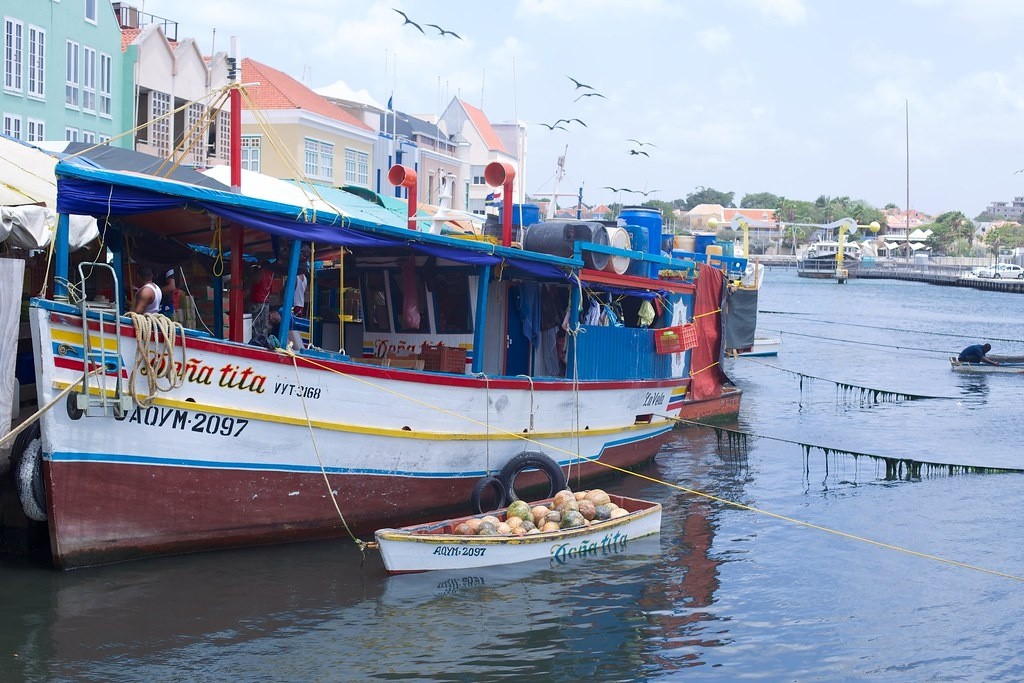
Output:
[{"x1": 972, "y1": 263, "x2": 1024, "y2": 280}]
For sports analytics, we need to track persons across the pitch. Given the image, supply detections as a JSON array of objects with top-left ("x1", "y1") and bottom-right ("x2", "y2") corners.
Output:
[
  {"x1": 130, "y1": 266, "x2": 162, "y2": 323},
  {"x1": 242, "y1": 262, "x2": 273, "y2": 347},
  {"x1": 283, "y1": 274, "x2": 308, "y2": 318},
  {"x1": 958, "y1": 343, "x2": 999, "y2": 367},
  {"x1": 151, "y1": 259, "x2": 176, "y2": 322}
]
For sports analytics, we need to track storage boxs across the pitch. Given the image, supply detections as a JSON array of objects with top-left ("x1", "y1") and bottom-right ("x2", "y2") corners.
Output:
[{"x1": 654, "y1": 322, "x2": 698, "y2": 355}]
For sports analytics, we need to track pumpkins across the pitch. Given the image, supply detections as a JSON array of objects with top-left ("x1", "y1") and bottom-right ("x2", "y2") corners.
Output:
[{"x1": 452, "y1": 490, "x2": 627, "y2": 536}]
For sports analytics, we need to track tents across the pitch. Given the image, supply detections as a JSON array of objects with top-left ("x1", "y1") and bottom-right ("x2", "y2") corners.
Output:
[{"x1": 0, "y1": 132, "x2": 485, "y2": 256}]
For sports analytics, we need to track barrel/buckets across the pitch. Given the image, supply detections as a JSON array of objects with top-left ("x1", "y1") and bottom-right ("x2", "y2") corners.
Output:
[{"x1": 520, "y1": 197, "x2": 735, "y2": 299}]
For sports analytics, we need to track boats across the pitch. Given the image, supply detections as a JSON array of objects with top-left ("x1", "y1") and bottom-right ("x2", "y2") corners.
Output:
[
  {"x1": 671, "y1": 385, "x2": 742, "y2": 429},
  {"x1": 719, "y1": 336, "x2": 783, "y2": 359},
  {"x1": 792, "y1": 235, "x2": 864, "y2": 272},
  {"x1": 26, "y1": 157, "x2": 702, "y2": 568},
  {"x1": 374, "y1": 493, "x2": 665, "y2": 578},
  {"x1": 951, "y1": 359, "x2": 1024, "y2": 374}
]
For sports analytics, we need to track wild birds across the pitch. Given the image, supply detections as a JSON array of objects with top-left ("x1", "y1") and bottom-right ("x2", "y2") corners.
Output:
[
  {"x1": 554, "y1": 118, "x2": 587, "y2": 128},
  {"x1": 626, "y1": 149, "x2": 650, "y2": 159},
  {"x1": 539, "y1": 123, "x2": 567, "y2": 131},
  {"x1": 632, "y1": 190, "x2": 660, "y2": 197},
  {"x1": 392, "y1": 7, "x2": 424, "y2": 35},
  {"x1": 425, "y1": 23, "x2": 462, "y2": 40},
  {"x1": 601, "y1": 186, "x2": 632, "y2": 193},
  {"x1": 626, "y1": 138, "x2": 656, "y2": 148},
  {"x1": 573, "y1": 93, "x2": 607, "y2": 103},
  {"x1": 567, "y1": 75, "x2": 594, "y2": 90}
]
